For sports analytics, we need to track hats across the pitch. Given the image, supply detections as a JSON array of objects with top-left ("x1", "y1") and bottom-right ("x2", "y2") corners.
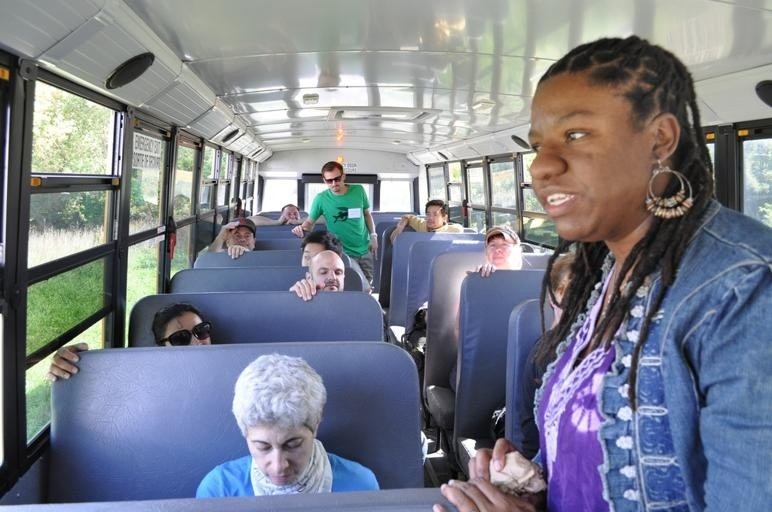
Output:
[
  {"x1": 229, "y1": 218, "x2": 256, "y2": 237},
  {"x1": 486, "y1": 225, "x2": 521, "y2": 245}
]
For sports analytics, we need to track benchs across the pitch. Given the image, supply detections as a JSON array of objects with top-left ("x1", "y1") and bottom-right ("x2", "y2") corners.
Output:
[
  {"x1": 126, "y1": 290, "x2": 385, "y2": 345},
  {"x1": 46, "y1": 342, "x2": 425, "y2": 504},
  {"x1": 0, "y1": 487, "x2": 459, "y2": 512},
  {"x1": 167, "y1": 210, "x2": 363, "y2": 292}
]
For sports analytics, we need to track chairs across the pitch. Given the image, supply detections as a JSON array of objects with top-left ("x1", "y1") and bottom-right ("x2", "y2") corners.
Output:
[{"x1": 373, "y1": 211, "x2": 566, "y2": 479}]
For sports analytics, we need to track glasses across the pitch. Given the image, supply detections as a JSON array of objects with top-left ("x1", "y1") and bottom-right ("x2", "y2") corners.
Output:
[
  {"x1": 161, "y1": 321, "x2": 213, "y2": 346},
  {"x1": 324, "y1": 174, "x2": 342, "y2": 184}
]
